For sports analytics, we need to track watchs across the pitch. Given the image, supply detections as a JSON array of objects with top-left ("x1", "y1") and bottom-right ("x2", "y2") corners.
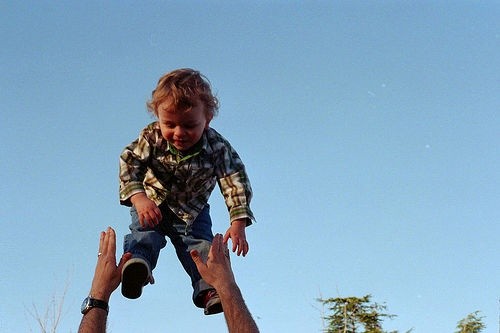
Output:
[{"x1": 80, "y1": 296, "x2": 110, "y2": 316}]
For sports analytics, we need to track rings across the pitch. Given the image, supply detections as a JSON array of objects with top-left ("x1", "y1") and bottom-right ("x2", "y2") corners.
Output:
[{"x1": 98, "y1": 253, "x2": 103, "y2": 257}]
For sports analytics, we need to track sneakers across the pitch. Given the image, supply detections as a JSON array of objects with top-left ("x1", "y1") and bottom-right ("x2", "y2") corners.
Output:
[
  {"x1": 204, "y1": 288, "x2": 223, "y2": 315},
  {"x1": 120, "y1": 257, "x2": 155, "y2": 300}
]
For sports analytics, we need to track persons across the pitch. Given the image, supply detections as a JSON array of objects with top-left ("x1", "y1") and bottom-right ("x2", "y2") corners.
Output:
[
  {"x1": 77, "y1": 225, "x2": 260, "y2": 333},
  {"x1": 118, "y1": 67, "x2": 259, "y2": 316}
]
